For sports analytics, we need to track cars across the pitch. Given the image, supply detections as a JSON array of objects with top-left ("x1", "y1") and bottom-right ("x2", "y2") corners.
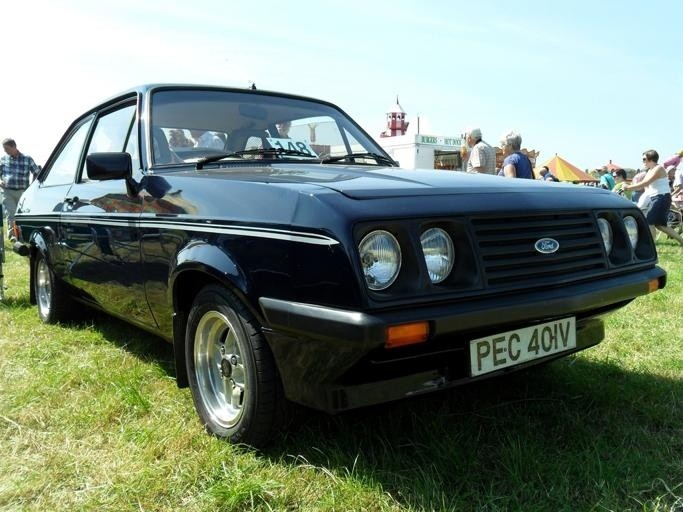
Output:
[{"x1": 13, "y1": 83, "x2": 667, "y2": 448}]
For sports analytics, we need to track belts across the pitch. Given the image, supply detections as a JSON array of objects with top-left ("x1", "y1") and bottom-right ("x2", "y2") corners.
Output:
[{"x1": 5, "y1": 186, "x2": 24, "y2": 192}]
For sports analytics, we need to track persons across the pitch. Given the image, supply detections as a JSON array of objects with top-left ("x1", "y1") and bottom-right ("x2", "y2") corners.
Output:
[
  {"x1": 460, "y1": 125, "x2": 496, "y2": 175},
  {"x1": 496, "y1": 128, "x2": 534, "y2": 180},
  {"x1": 167, "y1": 130, "x2": 194, "y2": 148},
  {"x1": 189, "y1": 128, "x2": 224, "y2": 151},
  {"x1": 594, "y1": 150, "x2": 682, "y2": 247},
  {"x1": 256, "y1": 120, "x2": 291, "y2": 159},
  {"x1": 538, "y1": 167, "x2": 552, "y2": 181},
  {"x1": 0, "y1": 138, "x2": 42, "y2": 244},
  {"x1": 543, "y1": 166, "x2": 556, "y2": 182},
  {"x1": 213, "y1": 131, "x2": 227, "y2": 142}
]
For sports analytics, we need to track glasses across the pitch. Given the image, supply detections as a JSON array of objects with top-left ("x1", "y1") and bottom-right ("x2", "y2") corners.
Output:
[{"x1": 642, "y1": 157, "x2": 648, "y2": 162}]
